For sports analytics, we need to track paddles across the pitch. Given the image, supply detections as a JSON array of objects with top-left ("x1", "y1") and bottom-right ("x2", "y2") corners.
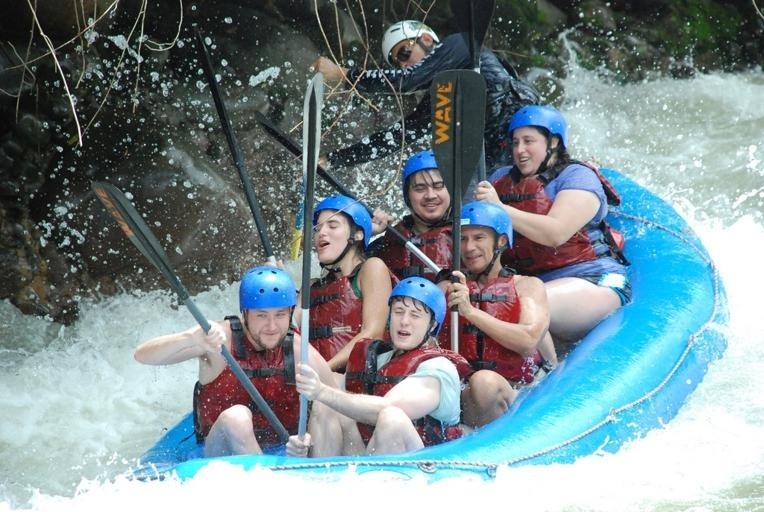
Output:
[
  {"x1": 255, "y1": 113, "x2": 443, "y2": 275},
  {"x1": 297, "y1": 73, "x2": 323, "y2": 440},
  {"x1": 451, "y1": 0, "x2": 494, "y2": 74},
  {"x1": 194, "y1": 24, "x2": 278, "y2": 267},
  {"x1": 430, "y1": 69, "x2": 488, "y2": 357},
  {"x1": 91, "y1": 181, "x2": 289, "y2": 441}
]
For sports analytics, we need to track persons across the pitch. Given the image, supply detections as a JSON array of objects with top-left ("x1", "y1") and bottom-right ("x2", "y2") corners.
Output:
[
  {"x1": 435, "y1": 200, "x2": 552, "y2": 430},
  {"x1": 305, "y1": 18, "x2": 543, "y2": 181},
  {"x1": 286, "y1": 275, "x2": 462, "y2": 456},
  {"x1": 469, "y1": 103, "x2": 633, "y2": 383},
  {"x1": 263, "y1": 193, "x2": 393, "y2": 388},
  {"x1": 369, "y1": 149, "x2": 454, "y2": 284},
  {"x1": 133, "y1": 264, "x2": 342, "y2": 456}
]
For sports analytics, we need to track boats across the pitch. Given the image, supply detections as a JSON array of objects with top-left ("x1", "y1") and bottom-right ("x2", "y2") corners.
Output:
[{"x1": 129, "y1": 169, "x2": 731, "y2": 487}]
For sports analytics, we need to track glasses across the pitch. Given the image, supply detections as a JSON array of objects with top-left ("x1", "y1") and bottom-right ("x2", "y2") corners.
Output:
[{"x1": 387, "y1": 39, "x2": 418, "y2": 68}]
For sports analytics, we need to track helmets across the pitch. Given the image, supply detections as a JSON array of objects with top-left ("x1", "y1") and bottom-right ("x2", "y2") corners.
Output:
[
  {"x1": 312, "y1": 194, "x2": 374, "y2": 252},
  {"x1": 389, "y1": 276, "x2": 448, "y2": 338},
  {"x1": 460, "y1": 201, "x2": 515, "y2": 251},
  {"x1": 505, "y1": 103, "x2": 570, "y2": 151},
  {"x1": 239, "y1": 265, "x2": 298, "y2": 313},
  {"x1": 402, "y1": 149, "x2": 439, "y2": 209},
  {"x1": 381, "y1": 19, "x2": 443, "y2": 68}
]
